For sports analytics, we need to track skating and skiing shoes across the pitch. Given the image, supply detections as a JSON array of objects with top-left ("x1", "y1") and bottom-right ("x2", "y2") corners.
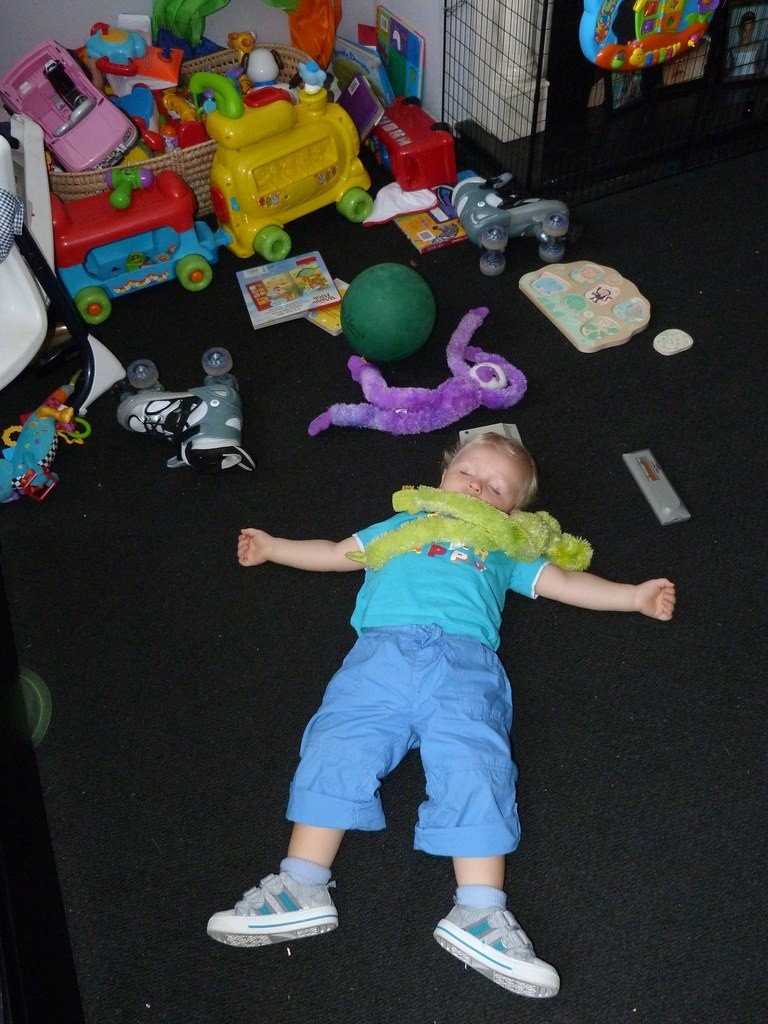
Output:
[
  {"x1": 439, "y1": 167, "x2": 570, "y2": 279},
  {"x1": 116, "y1": 345, "x2": 256, "y2": 476}
]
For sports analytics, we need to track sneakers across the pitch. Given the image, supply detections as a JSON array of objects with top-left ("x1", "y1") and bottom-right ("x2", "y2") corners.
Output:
[
  {"x1": 430, "y1": 899, "x2": 561, "y2": 1003},
  {"x1": 206, "y1": 872, "x2": 341, "y2": 951}
]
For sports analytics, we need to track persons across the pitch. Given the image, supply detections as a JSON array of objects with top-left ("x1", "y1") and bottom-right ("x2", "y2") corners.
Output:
[
  {"x1": 205, "y1": 431, "x2": 675, "y2": 997},
  {"x1": 663, "y1": 44, "x2": 705, "y2": 86},
  {"x1": 726, "y1": 11, "x2": 763, "y2": 76},
  {"x1": 615, "y1": 68, "x2": 638, "y2": 107}
]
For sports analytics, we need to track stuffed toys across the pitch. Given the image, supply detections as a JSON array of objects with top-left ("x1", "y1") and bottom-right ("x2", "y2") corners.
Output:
[
  {"x1": 352, "y1": 482, "x2": 595, "y2": 575},
  {"x1": 0, "y1": 382, "x2": 76, "y2": 502},
  {"x1": 309, "y1": 307, "x2": 529, "y2": 435}
]
